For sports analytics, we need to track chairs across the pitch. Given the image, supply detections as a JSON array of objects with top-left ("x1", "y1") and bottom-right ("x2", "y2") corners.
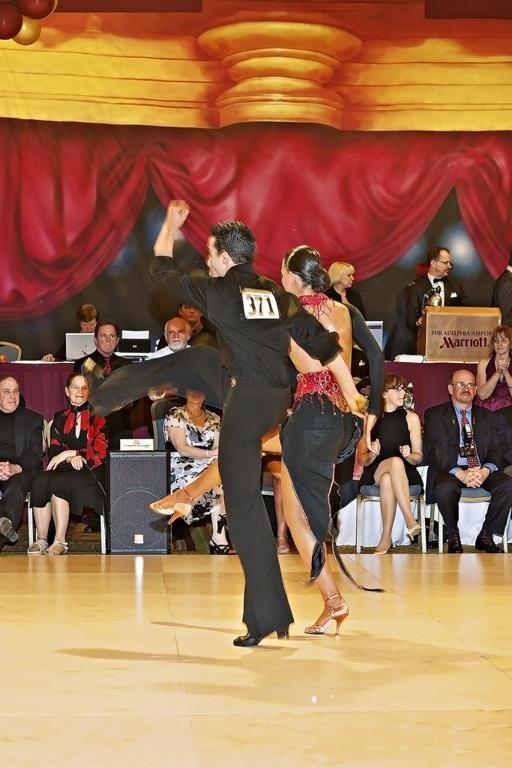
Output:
[
  {"x1": 150, "y1": 396, "x2": 223, "y2": 538},
  {"x1": 46, "y1": 420, "x2": 108, "y2": 554},
  {"x1": 355, "y1": 485, "x2": 427, "y2": 554},
  {"x1": 426, "y1": 485, "x2": 511, "y2": 553},
  {"x1": 0, "y1": 341, "x2": 23, "y2": 360},
  {"x1": 23, "y1": 418, "x2": 46, "y2": 547}
]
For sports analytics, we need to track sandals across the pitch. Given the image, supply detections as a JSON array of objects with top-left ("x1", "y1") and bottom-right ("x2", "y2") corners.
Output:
[
  {"x1": 27, "y1": 540, "x2": 49, "y2": 553},
  {"x1": 40, "y1": 540, "x2": 69, "y2": 556}
]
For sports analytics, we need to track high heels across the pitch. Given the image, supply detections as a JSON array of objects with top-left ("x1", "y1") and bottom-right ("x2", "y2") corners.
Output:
[
  {"x1": 150, "y1": 486, "x2": 421, "y2": 555},
  {"x1": 304, "y1": 593, "x2": 350, "y2": 635},
  {"x1": 233, "y1": 621, "x2": 289, "y2": 647}
]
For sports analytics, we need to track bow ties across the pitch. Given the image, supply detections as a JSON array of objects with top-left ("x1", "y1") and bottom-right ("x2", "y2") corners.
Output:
[{"x1": 433, "y1": 278, "x2": 446, "y2": 283}]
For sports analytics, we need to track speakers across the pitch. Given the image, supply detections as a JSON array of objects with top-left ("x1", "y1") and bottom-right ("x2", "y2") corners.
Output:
[{"x1": 109, "y1": 451, "x2": 170, "y2": 555}]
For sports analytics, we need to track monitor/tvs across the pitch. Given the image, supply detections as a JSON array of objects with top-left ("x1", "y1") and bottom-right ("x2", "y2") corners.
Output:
[{"x1": 65, "y1": 332, "x2": 97, "y2": 361}]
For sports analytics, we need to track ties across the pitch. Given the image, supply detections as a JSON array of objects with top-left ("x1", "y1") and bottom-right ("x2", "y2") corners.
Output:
[
  {"x1": 103, "y1": 356, "x2": 111, "y2": 378},
  {"x1": 460, "y1": 410, "x2": 480, "y2": 468}
]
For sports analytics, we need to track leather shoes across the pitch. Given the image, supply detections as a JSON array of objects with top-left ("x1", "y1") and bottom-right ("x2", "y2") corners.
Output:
[
  {"x1": 448, "y1": 527, "x2": 463, "y2": 554},
  {"x1": 0, "y1": 516, "x2": 18, "y2": 543},
  {"x1": 84, "y1": 526, "x2": 98, "y2": 534},
  {"x1": 475, "y1": 528, "x2": 505, "y2": 553}
]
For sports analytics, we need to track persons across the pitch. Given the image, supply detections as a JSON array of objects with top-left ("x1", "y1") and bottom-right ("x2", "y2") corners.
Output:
[
  {"x1": 81, "y1": 198, "x2": 369, "y2": 646},
  {"x1": 1, "y1": 248, "x2": 512, "y2": 553},
  {"x1": 149, "y1": 245, "x2": 384, "y2": 636}
]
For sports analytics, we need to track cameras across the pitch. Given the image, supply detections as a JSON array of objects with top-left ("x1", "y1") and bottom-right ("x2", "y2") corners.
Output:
[{"x1": 459, "y1": 423, "x2": 476, "y2": 458}]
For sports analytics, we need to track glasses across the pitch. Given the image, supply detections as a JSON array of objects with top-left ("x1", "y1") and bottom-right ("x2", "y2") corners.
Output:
[
  {"x1": 388, "y1": 386, "x2": 407, "y2": 392},
  {"x1": 434, "y1": 259, "x2": 455, "y2": 266},
  {"x1": 69, "y1": 385, "x2": 88, "y2": 393},
  {"x1": 452, "y1": 382, "x2": 477, "y2": 389}
]
